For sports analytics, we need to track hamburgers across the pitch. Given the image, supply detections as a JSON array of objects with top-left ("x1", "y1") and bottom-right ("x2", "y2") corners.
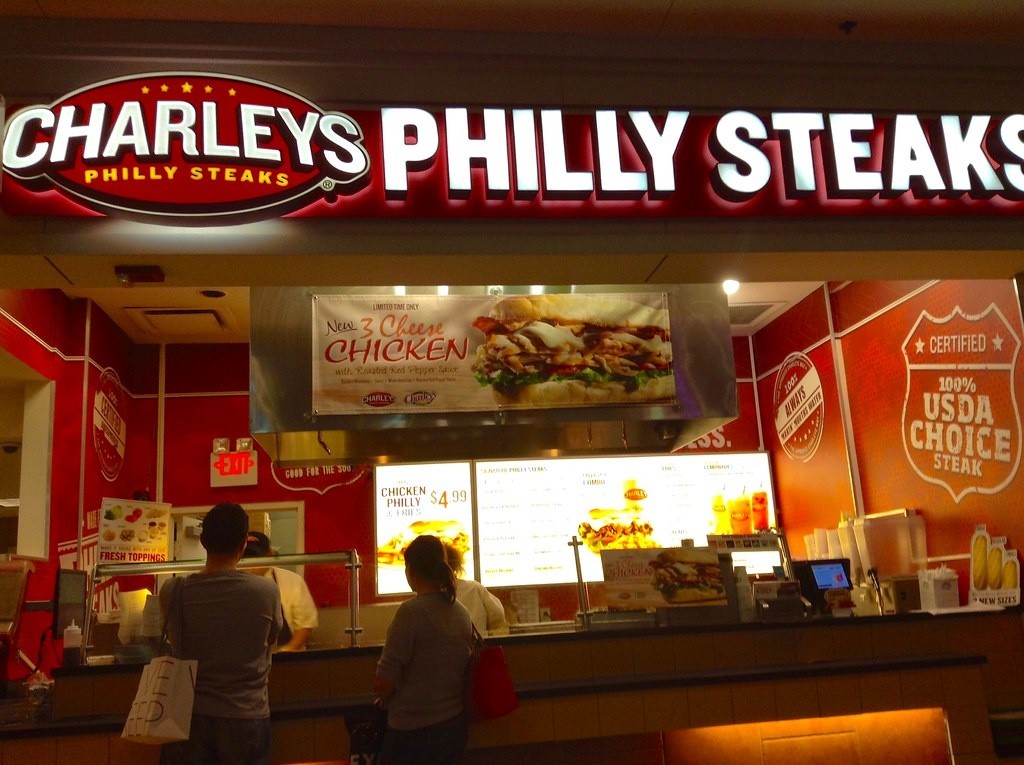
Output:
[
  {"x1": 469, "y1": 296, "x2": 675, "y2": 411},
  {"x1": 577, "y1": 509, "x2": 660, "y2": 554}
]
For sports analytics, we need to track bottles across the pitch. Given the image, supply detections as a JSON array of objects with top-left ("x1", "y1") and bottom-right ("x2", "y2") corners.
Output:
[{"x1": 62, "y1": 619, "x2": 81, "y2": 668}]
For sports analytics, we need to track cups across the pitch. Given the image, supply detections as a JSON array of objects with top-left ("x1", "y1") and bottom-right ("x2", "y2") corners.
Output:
[
  {"x1": 138, "y1": 521, "x2": 167, "y2": 542},
  {"x1": 805, "y1": 516, "x2": 872, "y2": 586},
  {"x1": 712, "y1": 491, "x2": 768, "y2": 535}
]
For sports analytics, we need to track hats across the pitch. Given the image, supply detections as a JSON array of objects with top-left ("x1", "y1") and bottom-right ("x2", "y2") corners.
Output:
[{"x1": 241, "y1": 532, "x2": 270, "y2": 558}]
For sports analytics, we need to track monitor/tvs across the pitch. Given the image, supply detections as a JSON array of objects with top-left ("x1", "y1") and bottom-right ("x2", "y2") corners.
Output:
[
  {"x1": 790, "y1": 558, "x2": 852, "y2": 615},
  {"x1": 53, "y1": 568, "x2": 88, "y2": 639}
]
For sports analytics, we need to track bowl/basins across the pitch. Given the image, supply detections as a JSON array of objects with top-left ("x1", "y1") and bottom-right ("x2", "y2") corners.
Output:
[
  {"x1": 114, "y1": 644, "x2": 151, "y2": 664},
  {"x1": 86, "y1": 654, "x2": 114, "y2": 665}
]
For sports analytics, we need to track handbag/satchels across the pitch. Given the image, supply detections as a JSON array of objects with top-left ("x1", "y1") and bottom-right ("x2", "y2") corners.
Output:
[
  {"x1": 463, "y1": 624, "x2": 517, "y2": 722},
  {"x1": 121, "y1": 576, "x2": 198, "y2": 745},
  {"x1": 344, "y1": 696, "x2": 385, "y2": 764}
]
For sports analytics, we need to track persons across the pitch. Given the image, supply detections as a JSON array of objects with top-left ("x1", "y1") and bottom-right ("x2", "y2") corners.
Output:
[
  {"x1": 375, "y1": 534, "x2": 509, "y2": 765},
  {"x1": 159, "y1": 503, "x2": 282, "y2": 765},
  {"x1": 236, "y1": 531, "x2": 318, "y2": 654}
]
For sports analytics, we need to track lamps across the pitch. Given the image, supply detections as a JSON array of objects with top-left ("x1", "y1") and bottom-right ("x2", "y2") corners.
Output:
[
  {"x1": 208, "y1": 437, "x2": 258, "y2": 487},
  {"x1": 114, "y1": 265, "x2": 165, "y2": 288}
]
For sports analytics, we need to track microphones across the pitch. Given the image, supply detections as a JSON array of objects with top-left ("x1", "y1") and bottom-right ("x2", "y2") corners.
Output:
[{"x1": 866, "y1": 568, "x2": 881, "y2": 592}]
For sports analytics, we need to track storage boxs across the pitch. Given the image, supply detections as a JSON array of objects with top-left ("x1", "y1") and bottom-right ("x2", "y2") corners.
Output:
[
  {"x1": 879, "y1": 576, "x2": 921, "y2": 615},
  {"x1": 917, "y1": 567, "x2": 961, "y2": 610}
]
